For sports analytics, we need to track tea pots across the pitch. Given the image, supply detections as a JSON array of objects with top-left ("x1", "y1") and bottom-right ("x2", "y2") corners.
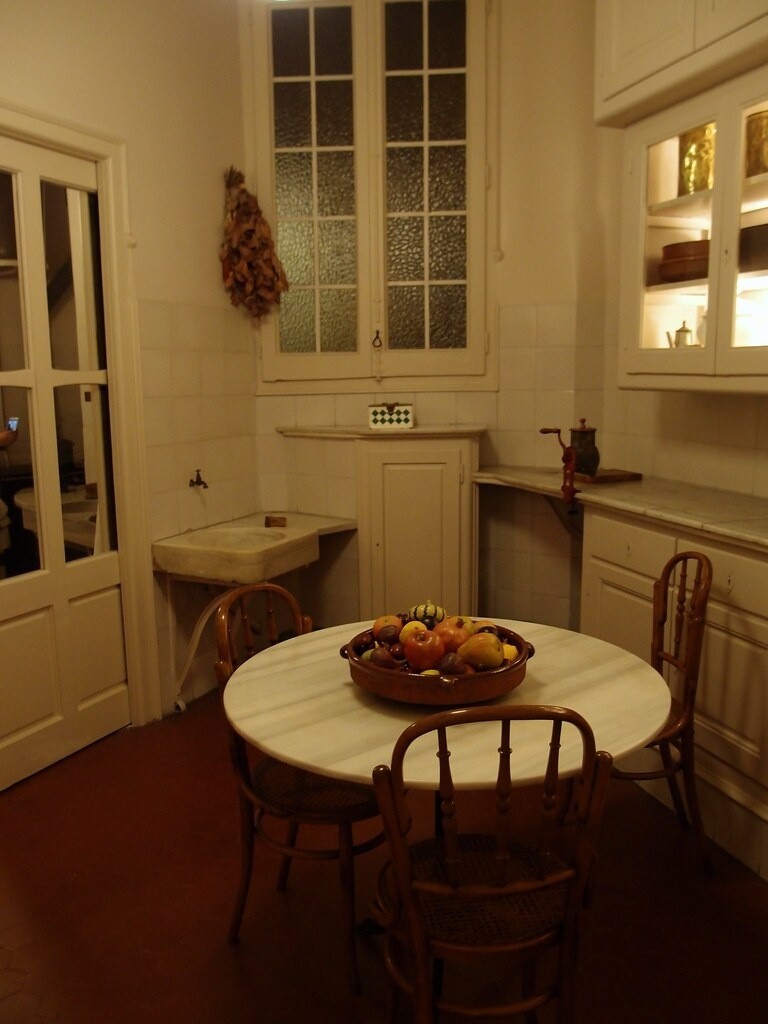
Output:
[
  {"x1": 666, "y1": 320, "x2": 694, "y2": 348},
  {"x1": 541, "y1": 418, "x2": 600, "y2": 477}
]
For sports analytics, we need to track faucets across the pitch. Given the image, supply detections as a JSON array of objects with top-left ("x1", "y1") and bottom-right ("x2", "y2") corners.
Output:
[{"x1": 189, "y1": 469, "x2": 209, "y2": 489}]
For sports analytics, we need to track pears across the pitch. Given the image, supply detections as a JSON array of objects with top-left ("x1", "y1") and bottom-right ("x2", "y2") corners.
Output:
[{"x1": 454, "y1": 632, "x2": 504, "y2": 670}]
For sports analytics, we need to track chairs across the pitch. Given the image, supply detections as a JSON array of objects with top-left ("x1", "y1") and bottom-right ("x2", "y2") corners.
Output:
[
  {"x1": 372, "y1": 704, "x2": 614, "y2": 1023},
  {"x1": 211, "y1": 582, "x2": 388, "y2": 987},
  {"x1": 611, "y1": 551, "x2": 717, "y2": 893}
]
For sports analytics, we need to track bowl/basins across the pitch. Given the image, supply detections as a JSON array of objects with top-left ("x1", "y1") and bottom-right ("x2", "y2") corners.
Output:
[
  {"x1": 662, "y1": 240, "x2": 710, "y2": 259},
  {"x1": 659, "y1": 256, "x2": 708, "y2": 282}
]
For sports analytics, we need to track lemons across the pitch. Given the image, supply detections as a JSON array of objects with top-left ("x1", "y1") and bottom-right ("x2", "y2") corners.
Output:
[
  {"x1": 502, "y1": 644, "x2": 521, "y2": 662},
  {"x1": 397, "y1": 620, "x2": 428, "y2": 644}
]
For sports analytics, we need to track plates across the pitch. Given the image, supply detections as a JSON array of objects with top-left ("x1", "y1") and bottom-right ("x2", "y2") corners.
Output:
[{"x1": 340, "y1": 621, "x2": 535, "y2": 705}]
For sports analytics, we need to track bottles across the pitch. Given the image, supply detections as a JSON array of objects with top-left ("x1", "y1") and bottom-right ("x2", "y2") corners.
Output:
[
  {"x1": 679, "y1": 125, "x2": 709, "y2": 197},
  {"x1": 743, "y1": 110, "x2": 768, "y2": 177}
]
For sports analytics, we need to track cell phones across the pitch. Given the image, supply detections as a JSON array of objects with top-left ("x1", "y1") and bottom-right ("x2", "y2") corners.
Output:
[{"x1": 7, "y1": 417, "x2": 19, "y2": 431}]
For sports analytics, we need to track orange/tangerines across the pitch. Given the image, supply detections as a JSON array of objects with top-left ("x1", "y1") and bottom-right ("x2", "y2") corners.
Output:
[{"x1": 373, "y1": 615, "x2": 403, "y2": 636}]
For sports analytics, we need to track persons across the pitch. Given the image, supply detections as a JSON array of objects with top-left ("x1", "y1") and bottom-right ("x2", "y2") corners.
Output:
[{"x1": 0, "y1": 428, "x2": 19, "y2": 449}]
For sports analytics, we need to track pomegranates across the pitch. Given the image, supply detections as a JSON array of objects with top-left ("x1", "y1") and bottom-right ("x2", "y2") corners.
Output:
[
  {"x1": 404, "y1": 630, "x2": 445, "y2": 670},
  {"x1": 432, "y1": 615, "x2": 496, "y2": 651}
]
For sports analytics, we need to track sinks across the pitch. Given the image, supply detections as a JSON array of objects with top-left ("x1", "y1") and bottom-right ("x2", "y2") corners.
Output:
[{"x1": 151, "y1": 523, "x2": 320, "y2": 587}]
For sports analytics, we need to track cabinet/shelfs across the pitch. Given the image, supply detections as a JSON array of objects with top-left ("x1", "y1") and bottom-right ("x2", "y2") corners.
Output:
[
  {"x1": 591, "y1": 0, "x2": 768, "y2": 128},
  {"x1": 275, "y1": 426, "x2": 488, "y2": 631},
  {"x1": 617, "y1": 64, "x2": 768, "y2": 394},
  {"x1": 578, "y1": 509, "x2": 768, "y2": 817}
]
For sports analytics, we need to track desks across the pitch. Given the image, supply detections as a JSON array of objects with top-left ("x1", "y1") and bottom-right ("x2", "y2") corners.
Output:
[{"x1": 218, "y1": 615, "x2": 671, "y2": 1023}]
